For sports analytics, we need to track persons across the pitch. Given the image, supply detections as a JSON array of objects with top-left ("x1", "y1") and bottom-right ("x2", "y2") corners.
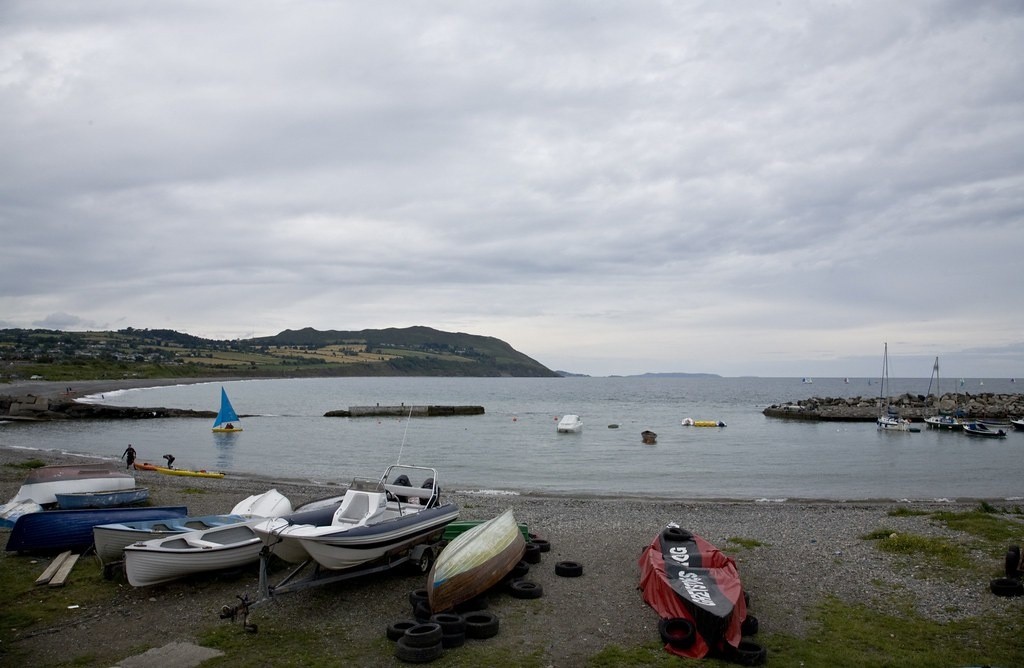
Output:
[
  {"x1": 163, "y1": 454, "x2": 175, "y2": 469},
  {"x1": 121, "y1": 444, "x2": 137, "y2": 470},
  {"x1": 225, "y1": 423, "x2": 234, "y2": 429}
]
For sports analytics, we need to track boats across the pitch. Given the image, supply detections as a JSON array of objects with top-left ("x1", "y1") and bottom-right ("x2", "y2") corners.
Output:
[
  {"x1": 425, "y1": 505, "x2": 527, "y2": 617},
  {"x1": 1010, "y1": 419, "x2": 1024, "y2": 429},
  {"x1": 134, "y1": 462, "x2": 225, "y2": 479},
  {"x1": 231, "y1": 488, "x2": 295, "y2": 530},
  {"x1": 649, "y1": 520, "x2": 743, "y2": 638},
  {"x1": 0, "y1": 461, "x2": 136, "y2": 528},
  {"x1": 55, "y1": 487, "x2": 151, "y2": 509},
  {"x1": 251, "y1": 403, "x2": 461, "y2": 572},
  {"x1": 122, "y1": 516, "x2": 280, "y2": 588},
  {"x1": 91, "y1": 513, "x2": 263, "y2": 577},
  {"x1": 962, "y1": 423, "x2": 1008, "y2": 436},
  {"x1": 5, "y1": 506, "x2": 188, "y2": 561},
  {"x1": 557, "y1": 414, "x2": 582, "y2": 434}
]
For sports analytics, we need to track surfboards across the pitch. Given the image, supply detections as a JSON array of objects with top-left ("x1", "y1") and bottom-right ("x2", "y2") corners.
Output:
[{"x1": 134, "y1": 462, "x2": 227, "y2": 478}]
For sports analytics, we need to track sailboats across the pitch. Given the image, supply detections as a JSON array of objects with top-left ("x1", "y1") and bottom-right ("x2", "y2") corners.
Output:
[
  {"x1": 877, "y1": 342, "x2": 911, "y2": 431},
  {"x1": 922, "y1": 357, "x2": 968, "y2": 428},
  {"x1": 211, "y1": 386, "x2": 243, "y2": 432}
]
